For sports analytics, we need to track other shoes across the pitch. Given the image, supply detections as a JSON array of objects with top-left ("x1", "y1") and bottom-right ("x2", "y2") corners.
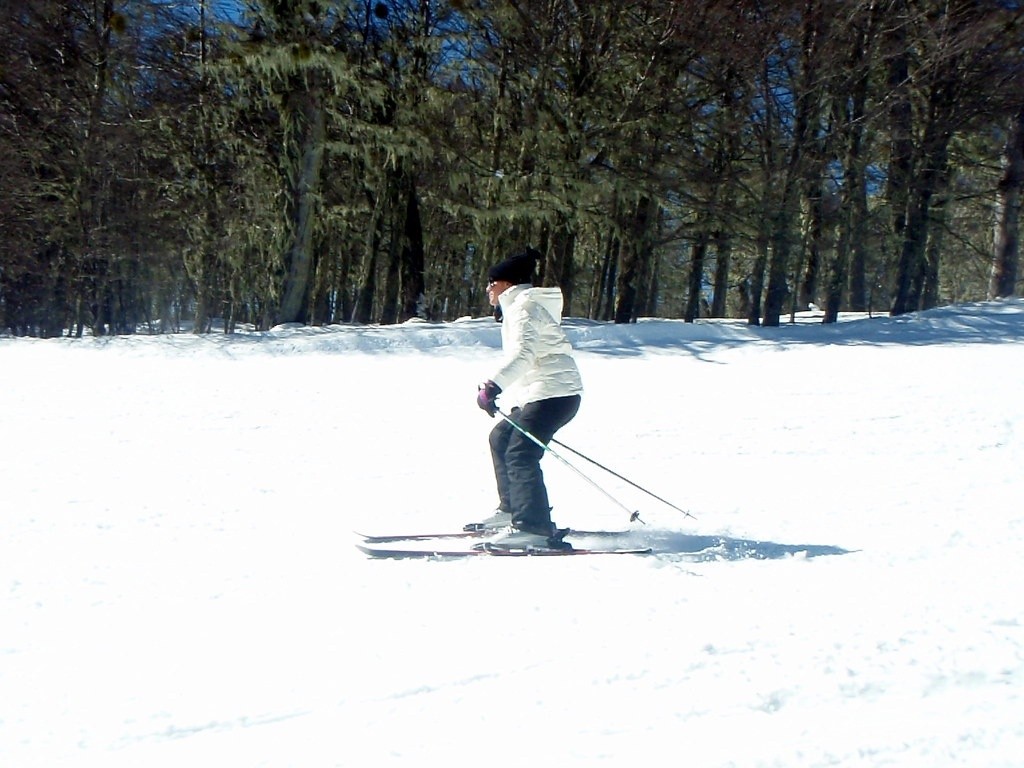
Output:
[
  {"x1": 496, "y1": 527, "x2": 572, "y2": 549},
  {"x1": 483, "y1": 510, "x2": 511, "y2": 526}
]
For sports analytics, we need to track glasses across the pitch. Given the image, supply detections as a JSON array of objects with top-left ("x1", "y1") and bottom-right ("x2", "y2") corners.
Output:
[{"x1": 489, "y1": 279, "x2": 495, "y2": 287}]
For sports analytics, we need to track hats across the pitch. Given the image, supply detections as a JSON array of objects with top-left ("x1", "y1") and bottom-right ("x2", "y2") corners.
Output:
[{"x1": 488, "y1": 246, "x2": 541, "y2": 285}]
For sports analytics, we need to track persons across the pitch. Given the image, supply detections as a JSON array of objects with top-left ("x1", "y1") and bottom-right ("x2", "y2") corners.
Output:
[{"x1": 464, "y1": 254, "x2": 585, "y2": 553}]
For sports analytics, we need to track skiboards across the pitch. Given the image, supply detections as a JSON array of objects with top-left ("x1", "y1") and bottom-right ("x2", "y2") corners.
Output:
[{"x1": 358, "y1": 529, "x2": 655, "y2": 559}]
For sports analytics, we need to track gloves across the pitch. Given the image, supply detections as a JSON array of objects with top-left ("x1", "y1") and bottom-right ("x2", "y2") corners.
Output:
[{"x1": 477, "y1": 379, "x2": 502, "y2": 418}]
